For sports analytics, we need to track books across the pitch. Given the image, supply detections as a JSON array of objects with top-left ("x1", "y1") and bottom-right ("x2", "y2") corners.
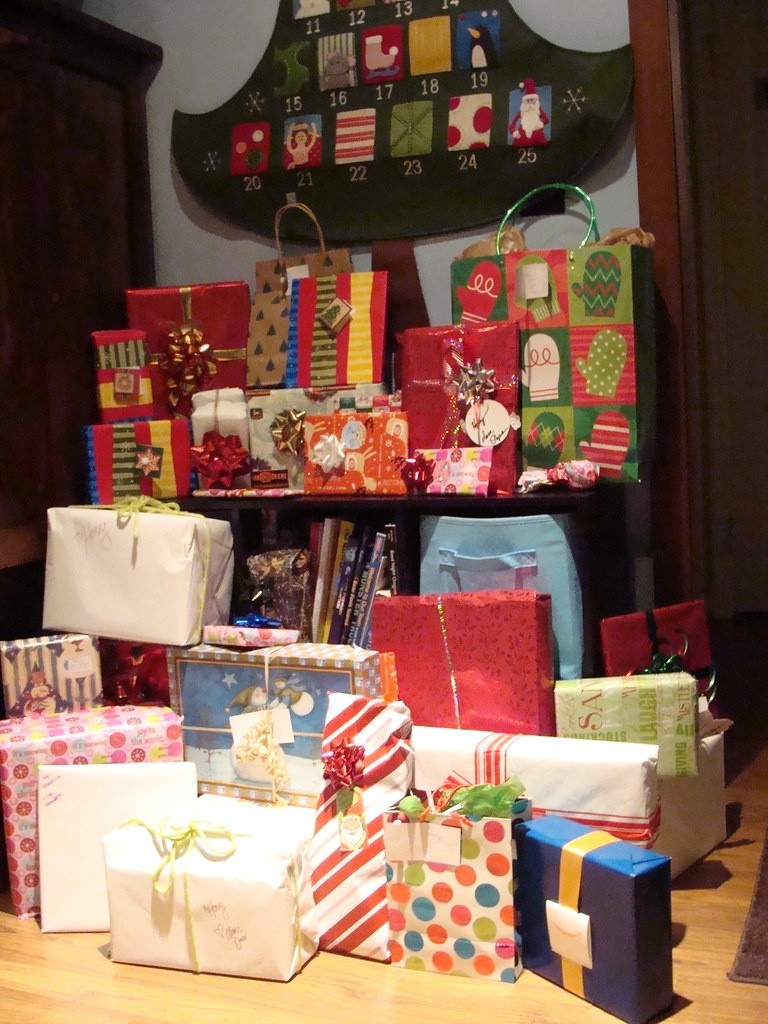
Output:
[{"x1": 301, "y1": 516, "x2": 400, "y2": 651}]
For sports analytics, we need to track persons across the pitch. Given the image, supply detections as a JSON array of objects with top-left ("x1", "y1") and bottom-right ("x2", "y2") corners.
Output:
[{"x1": 293, "y1": 548, "x2": 310, "y2": 589}]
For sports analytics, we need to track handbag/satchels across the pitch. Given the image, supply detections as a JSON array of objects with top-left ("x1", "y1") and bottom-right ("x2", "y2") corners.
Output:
[
  {"x1": 447, "y1": 183, "x2": 656, "y2": 483},
  {"x1": 245, "y1": 202, "x2": 355, "y2": 389}
]
